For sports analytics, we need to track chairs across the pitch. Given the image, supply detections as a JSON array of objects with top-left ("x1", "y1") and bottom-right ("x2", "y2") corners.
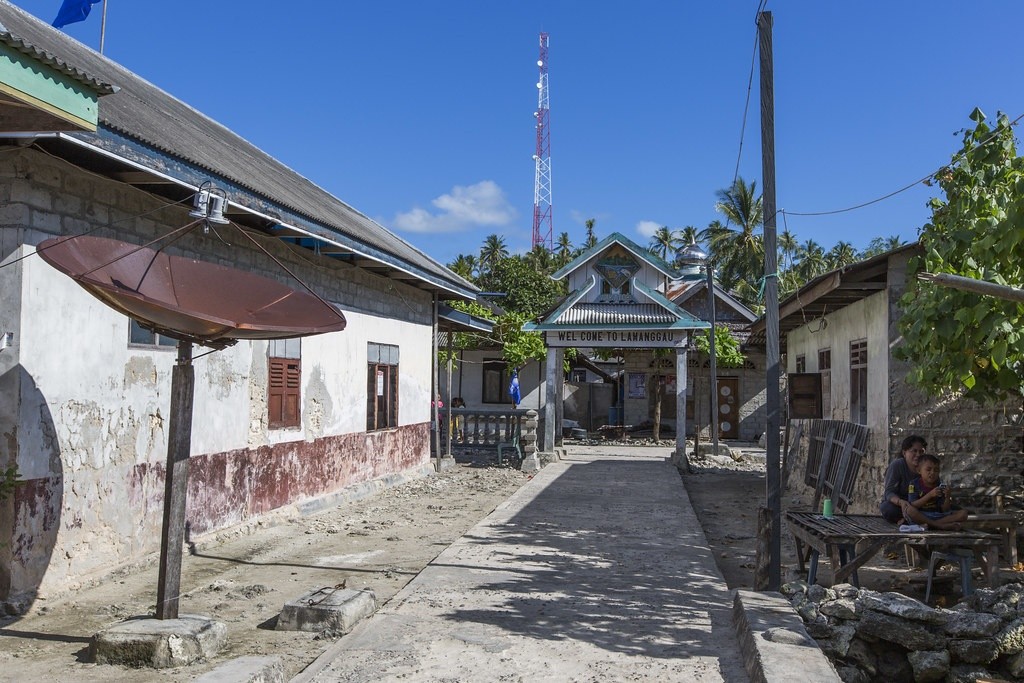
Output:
[{"x1": 497, "y1": 422, "x2": 523, "y2": 468}]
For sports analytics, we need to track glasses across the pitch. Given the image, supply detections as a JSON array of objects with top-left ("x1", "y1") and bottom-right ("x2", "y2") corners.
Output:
[{"x1": 907, "y1": 448, "x2": 925, "y2": 454}]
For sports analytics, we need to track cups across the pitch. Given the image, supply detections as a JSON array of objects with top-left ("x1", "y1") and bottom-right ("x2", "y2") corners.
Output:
[{"x1": 823, "y1": 495, "x2": 833, "y2": 518}]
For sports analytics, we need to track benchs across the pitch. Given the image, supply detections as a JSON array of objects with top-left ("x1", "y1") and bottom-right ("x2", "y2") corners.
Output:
[{"x1": 784, "y1": 510, "x2": 1003, "y2": 606}]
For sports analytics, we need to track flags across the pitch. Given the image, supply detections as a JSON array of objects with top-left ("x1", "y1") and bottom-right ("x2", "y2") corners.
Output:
[{"x1": 506, "y1": 367, "x2": 520, "y2": 405}]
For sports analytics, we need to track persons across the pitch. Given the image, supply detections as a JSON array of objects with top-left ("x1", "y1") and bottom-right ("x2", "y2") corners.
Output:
[
  {"x1": 878, "y1": 434, "x2": 928, "y2": 525},
  {"x1": 450, "y1": 397, "x2": 458, "y2": 440},
  {"x1": 905, "y1": 454, "x2": 968, "y2": 531},
  {"x1": 456, "y1": 397, "x2": 466, "y2": 442},
  {"x1": 431, "y1": 394, "x2": 444, "y2": 441}
]
